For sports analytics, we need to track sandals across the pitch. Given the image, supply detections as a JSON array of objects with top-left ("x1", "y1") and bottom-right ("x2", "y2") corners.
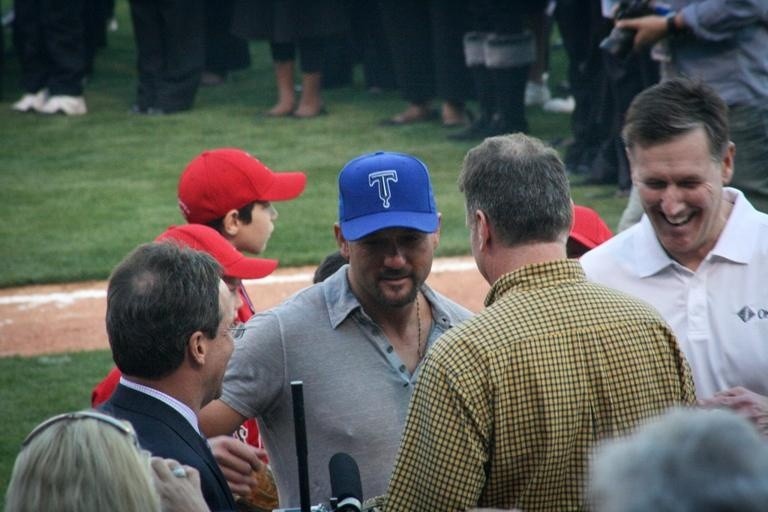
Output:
[
  {"x1": 291, "y1": 109, "x2": 328, "y2": 120},
  {"x1": 264, "y1": 105, "x2": 294, "y2": 118},
  {"x1": 442, "y1": 113, "x2": 470, "y2": 129},
  {"x1": 376, "y1": 108, "x2": 434, "y2": 127}
]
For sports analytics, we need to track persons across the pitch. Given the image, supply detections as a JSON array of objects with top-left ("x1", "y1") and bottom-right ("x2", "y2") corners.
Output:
[
  {"x1": 177, "y1": 145, "x2": 306, "y2": 323},
  {"x1": 89, "y1": 238, "x2": 247, "y2": 512},
  {"x1": 566, "y1": 197, "x2": 613, "y2": 259},
  {"x1": 579, "y1": 76, "x2": 768, "y2": 438},
  {"x1": 362, "y1": 132, "x2": 697, "y2": 511},
  {"x1": 6, "y1": 407, "x2": 210, "y2": 512},
  {"x1": 585, "y1": 408, "x2": 768, "y2": 511},
  {"x1": 314, "y1": 249, "x2": 350, "y2": 285},
  {"x1": 93, "y1": 222, "x2": 278, "y2": 466},
  {"x1": 195, "y1": 151, "x2": 480, "y2": 512}
]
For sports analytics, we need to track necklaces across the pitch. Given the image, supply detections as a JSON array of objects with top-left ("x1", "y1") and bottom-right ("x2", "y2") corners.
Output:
[{"x1": 415, "y1": 295, "x2": 424, "y2": 360}]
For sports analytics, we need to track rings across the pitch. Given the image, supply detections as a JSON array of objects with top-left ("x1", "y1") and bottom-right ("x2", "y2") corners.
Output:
[{"x1": 173, "y1": 468, "x2": 186, "y2": 477}]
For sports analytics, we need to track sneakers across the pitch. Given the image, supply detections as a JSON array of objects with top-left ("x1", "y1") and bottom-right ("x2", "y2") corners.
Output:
[
  {"x1": 10, "y1": 87, "x2": 46, "y2": 111},
  {"x1": 43, "y1": 95, "x2": 87, "y2": 117}
]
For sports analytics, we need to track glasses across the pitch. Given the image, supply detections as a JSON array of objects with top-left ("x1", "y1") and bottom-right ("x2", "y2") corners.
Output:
[
  {"x1": 22, "y1": 410, "x2": 141, "y2": 447},
  {"x1": 214, "y1": 322, "x2": 246, "y2": 340}
]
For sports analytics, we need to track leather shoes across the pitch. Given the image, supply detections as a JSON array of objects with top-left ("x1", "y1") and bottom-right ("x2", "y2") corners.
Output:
[
  {"x1": 130, "y1": 103, "x2": 155, "y2": 113},
  {"x1": 445, "y1": 115, "x2": 489, "y2": 141},
  {"x1": 484, "y1": 115, "x2": 529, "y2": 137},
  {"x1": 148, "y1": 105, "x2": 191, "y2": 118}
]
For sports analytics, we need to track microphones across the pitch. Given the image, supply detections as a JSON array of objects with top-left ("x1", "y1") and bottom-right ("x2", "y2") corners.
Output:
[{"x1": 327, "y1": 451, "x2": 363, "y2": 512}]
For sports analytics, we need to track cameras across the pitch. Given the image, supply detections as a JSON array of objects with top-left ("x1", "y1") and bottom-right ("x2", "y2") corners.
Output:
[{"x1": 597, "y1": 2, "x2": 661, "y2": 71}]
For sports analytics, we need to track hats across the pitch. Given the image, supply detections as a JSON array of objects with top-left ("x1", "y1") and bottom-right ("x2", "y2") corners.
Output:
[
  {"x1": 569, "y1": 206, "x2": 613, "y2": 250},
  {"x1": 179, "y1": 149, "x2": 306, "y2": 225},
  {"x1": 153, "y1": 223, "x2": 278, "y2": 280},
  {"x1": 336, "y1": 153, "x2": 438, "y2": 243}
]
